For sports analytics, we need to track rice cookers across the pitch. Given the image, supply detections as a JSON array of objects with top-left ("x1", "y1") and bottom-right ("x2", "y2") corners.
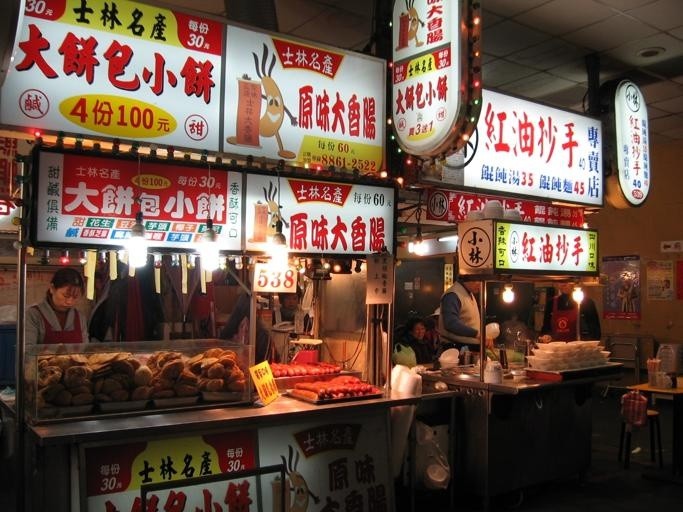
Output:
[{"x1": 272, "y1": 321, "x2": 295, "y2": 354}]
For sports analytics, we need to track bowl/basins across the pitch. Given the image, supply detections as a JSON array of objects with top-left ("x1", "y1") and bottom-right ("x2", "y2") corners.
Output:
[
  {"x1": 484, "y1": 362, "x2": 502, "y2": 384},
  {"x1": 438, "y1": 348, "x2": 459, "y2": 368}
]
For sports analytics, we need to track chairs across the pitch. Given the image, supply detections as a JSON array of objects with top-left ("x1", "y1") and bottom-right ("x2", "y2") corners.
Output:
[{"x1": 617, "y1": 389, "x2": 662, "y2": 472}]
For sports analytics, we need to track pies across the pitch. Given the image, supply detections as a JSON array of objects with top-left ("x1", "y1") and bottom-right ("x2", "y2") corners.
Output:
[{"x1": 25, "y1": 347, "x2": 247, "y2": 409}]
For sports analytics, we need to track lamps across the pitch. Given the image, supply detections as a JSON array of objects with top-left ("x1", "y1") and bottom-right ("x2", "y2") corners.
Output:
[
  {"x1": 568, "y1": 282, "x2": 585, "y2": 306},
  {"x1": 119, "y1": 148, "x2": 149, "y2": 267},
  {"x1": 499, "y1": 281, "x2": 517, "y2": 304},
  {"x1": 196, "y1": 155, "x2": 230, "y2": 278}
]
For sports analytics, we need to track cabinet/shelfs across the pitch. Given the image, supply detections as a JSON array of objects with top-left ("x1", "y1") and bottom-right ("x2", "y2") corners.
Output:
[{"x1": 23, "y1": 336, "x2": 252, "y2": 424}]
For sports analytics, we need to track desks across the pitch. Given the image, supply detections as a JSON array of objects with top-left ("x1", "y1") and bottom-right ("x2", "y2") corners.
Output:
[{"x1": 627, "y1": 359, "x2": 683, "y2": 479}]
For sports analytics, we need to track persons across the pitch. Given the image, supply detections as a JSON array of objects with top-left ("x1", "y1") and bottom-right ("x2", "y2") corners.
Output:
[
  {"x1": 438, "y1": 274, "x2": 494, "y2": 365},
  {"x1": 221, "y1": 292, "x2": 250, "y2": 343},
  {"x1": 272, "y1": 285, "x2": 312, "y2": 332},
  {"x1": 538, "y1": 285, "x2": 601, "y2": 343},
  {"x1": 25, "y1": 268, "x2": 89, "y2": 344},
  {"x1": 400, "y1": 319, "x2": 435, "y2": 364}
]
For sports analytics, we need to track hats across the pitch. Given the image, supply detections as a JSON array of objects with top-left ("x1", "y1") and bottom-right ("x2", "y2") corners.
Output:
[{"x1": 431, "y1": 307, "x2": 440, "y2": 316}]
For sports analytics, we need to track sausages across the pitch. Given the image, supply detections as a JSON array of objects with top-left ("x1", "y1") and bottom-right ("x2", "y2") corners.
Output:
[
  {"x1": 270, "y1": 362, "x2": 373, "y2": 403},
  {"x1": 289, "y1": 472, "x2": 309, "y2": 512},
  {"x1": 266, "y1": 200, "x2": 281, "y2": 237},
  {"x1": 407, "y1": 7, "x2": 419, "y2": 41},
  {"x1": 259, "y1": 76, "x2": 285, "y2": 137}
]
